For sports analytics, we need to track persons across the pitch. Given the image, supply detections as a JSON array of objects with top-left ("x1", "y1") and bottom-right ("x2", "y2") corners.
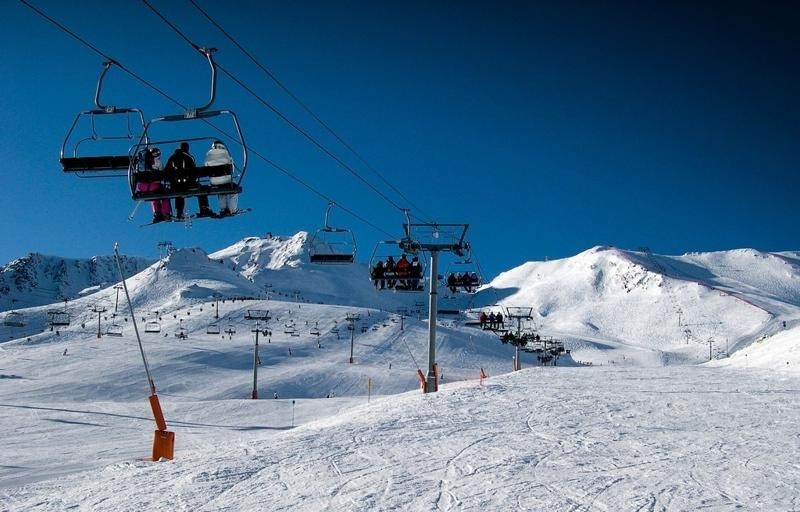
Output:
[
  {"x1": 372, "y1": 261, "x2": 385, "y2": 289},
  {"x1": 470, "y1": 272, "x2": 479, "y2": 292},
  {"x1": 205, "y1": 140, "x2": 238, "y2": 215},
  {"x1": 456, "y1": 272, "x2": 463, "y2": 293},
  {"x1": 447, "y1": 273, "x2": 457, "y2": 293},
  {"x1": 383, "y1": 256, "x2": 398, "y2": 289},
  {"x1": 163, "y1": 143, "x2": 210, "y2": 219},
  {"x1": 463, "y1": 271, "x2": 470, "y2": 293},
  {"x1": 410, "y1": 257, "x2": 421, "y2": 288},
  {"x1": 396, "y1": 254, "x2": 410, "y2": 286},
  {"x1": 138, "y1": 147, "x2": 171, "y2": 223},
  {"x1": 480, "y1": 311, "x2": 560, "y2": 363}
]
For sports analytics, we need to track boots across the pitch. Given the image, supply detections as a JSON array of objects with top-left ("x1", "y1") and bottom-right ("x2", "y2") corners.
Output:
[
  {"x1": 200, "y1": 205, "x2": 212, "y2": 216},
  {"x1": 220, "y1": 208, "x2": 240, "y2": 216},
  {"x1": 175, "y1": 209, "x2": 186, "y2": 219},
  {"x1": 153, "y1": 212, "x2": 172, "y2": 222}
]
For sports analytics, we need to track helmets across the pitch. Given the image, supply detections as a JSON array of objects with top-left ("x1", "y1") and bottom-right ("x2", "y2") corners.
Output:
[
  {"x1": 150, "y1": 148, "x2": 161, "y2": 157},
  {"x1": 181, "y1": 143, "x2": 189, "y2": 149}
]
